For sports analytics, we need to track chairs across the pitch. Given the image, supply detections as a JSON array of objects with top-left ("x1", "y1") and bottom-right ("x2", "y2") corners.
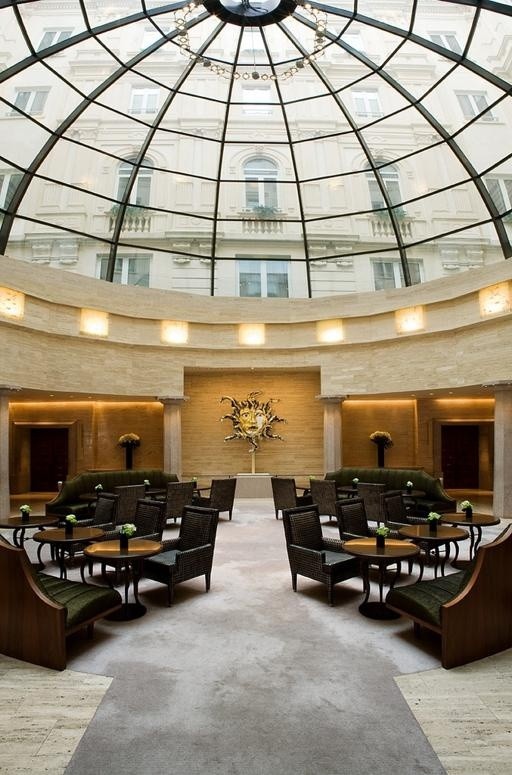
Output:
[
  {"x1": 352, "y1": 481, "x2": 408, "y2": 525},
  {"x1": 191, "y1": 477, "x2": 239, "y2": 522},
  {"x1": 96, "y1": 498, "x2": 170, "y2": 590},
  {"x1": 130, "y1": 504, "x2": 220, "y2": 611},
  {"x1": 382, "y1": 494, "x2": 451, "y2": 568},
  {"x1": 270, "y1": 475, "x2": 314, "y2": 520},
  {"x1": 280, "y1": 503, "x2": 367, "y2": 608},
  {"x1": 102, "y1": 482, "x2": 151, "y2": 524},
  {"x1": 154, "y1": 480, "x2": 197, "y2": 525},
  {"x1": 308, "y1": 478, "x2": 357, "y2": 527},
  {"x1": 334, "y1": 496, "x2": 415, "y2": 587},
  {"x1": 57, "y1": 492, "x2": 120, "y2": 574}
]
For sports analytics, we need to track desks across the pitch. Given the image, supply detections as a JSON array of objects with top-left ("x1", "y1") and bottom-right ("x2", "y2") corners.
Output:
[
  {"x1": 398, "y1": 523, "x2": 470, "y2": 585},
  {"x1": 340, "y1": 535, "x2": 422, "y2": 621},
  {"x1": 0, "y1": 515, "x2": 60, "y2": 573},
  {"x1": 438, "y1": 512, "x2": 501, "y2": 572},
  {"x1": 81, "y1": 537, "x2": 163, "y2": 622},
  {"x1": 31, "y1": 523, "x2": 106, "y2": 584}
]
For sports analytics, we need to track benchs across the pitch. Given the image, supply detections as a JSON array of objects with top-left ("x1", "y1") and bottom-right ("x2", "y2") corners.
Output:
[
  {"x1": 384, "y1": 523, "x2": 511, "y2": 670},
  {"x1": 0, "y1": 534, "x2": 122, "y2": 671}
]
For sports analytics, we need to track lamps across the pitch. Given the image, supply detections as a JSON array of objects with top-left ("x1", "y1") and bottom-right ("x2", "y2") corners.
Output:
[{"x1": 173, "y1": 0, "x2": 328, "y2": 81}]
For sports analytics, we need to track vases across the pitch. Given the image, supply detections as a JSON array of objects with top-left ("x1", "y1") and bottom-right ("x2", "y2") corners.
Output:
[
  {"x1": 465, "y1": 507, "x2": 473, "y2": 518},
  {"x1": 429, "y1": 520, "x2": 438, "y2": 532},
  {"x1": 124, "y1": 448, "x2": 134, "y2": 470},
  {"x1": 119, "y1": 534, "x2": 129, "y2": 549},
  {"x1": 377, "y1": 445, "x2": 387, "y2": 468},
  {"x1": 376, "y1": 534, "x2": 386, "y2": 548},
  {"x1": 64, "y1": 520, "x2": 74, "y2": 535},
  {"x1": 21, "y1": 512, "x2": 30, "y2": 522}
]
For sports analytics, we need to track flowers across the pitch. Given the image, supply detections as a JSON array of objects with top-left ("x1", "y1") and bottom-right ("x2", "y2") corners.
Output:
[
  {"x1": 461, "y1": 500, "x2": 474, "y2": 512},
  {"x1": 65, "y1": 514, "x2": 78, "y2": 525},
  {"x1": 119, "y1": 522, "x2": 138, "y2": 537},
  {"x1": 19, "y1": 504, "x2": 32, "y2": 514},
  {"x1": 375, "y1": 522, "x2": 390, "y2": 537},
  {"x1": 369, "y1": 429, "x2": 395, "y2": 450},
  {"x1": 117, "y1": 432, "x2": 142, "y2": 449},
  {"x1": 427, "y1": 511, "x2": 442, "y2": 521}
]
